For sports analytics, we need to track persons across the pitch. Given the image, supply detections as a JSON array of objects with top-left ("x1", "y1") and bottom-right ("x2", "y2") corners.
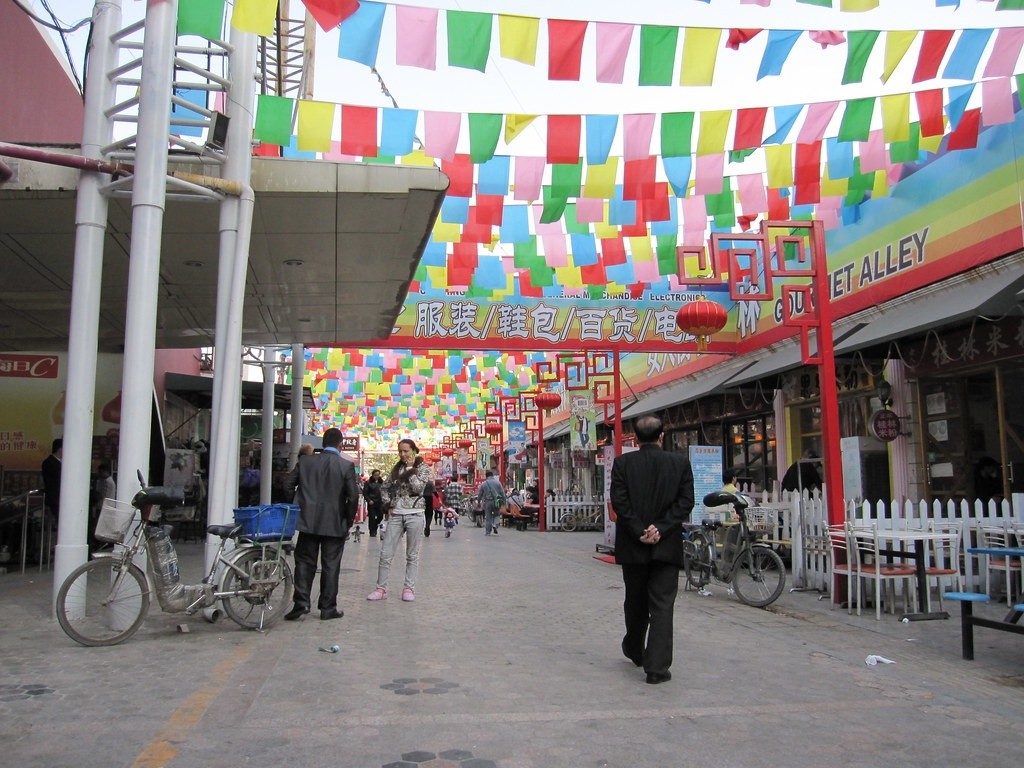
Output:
[
  {"x1": 610, "y1": 415, "x2": 694, "y2": 684},
  {"x1": 528, "y1": 478, "x2": 539, "y2": 505},
  {"x1": 363, "y1": 470, "x2": 384, "y2": 536},
  {"x1": 282, "y1": 428, "x2": 359, "y2": 622},
  {"x1": 422, "y1": 477, "x2": 464, "y2": 538},
  {"x1": 42, "y1": 436, "x2": 62, "y2": 524},
  {"x1": 508, "y1": 487, "x2": 525, "y2": 512},
  {"x1": 478, "y1": 471, "x2": 507, "y2": 536},
  {"x1": 93, "y1": 464, "x2": 116, "y2": 553},
  {"x1": 782, "y1": 450, "x2": 824, "y2": 499},
  {"x1": 365, "y1": 438, "x2": 431, "y2": 602}
]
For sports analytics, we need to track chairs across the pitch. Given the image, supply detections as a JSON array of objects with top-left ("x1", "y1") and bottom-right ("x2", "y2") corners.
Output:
[
  {"x1": 826, "y1": 518, "x2": 1024, "y2": 660},
  {"x1": 165, "y1": 474, "x2": 204, "y2": 544}
]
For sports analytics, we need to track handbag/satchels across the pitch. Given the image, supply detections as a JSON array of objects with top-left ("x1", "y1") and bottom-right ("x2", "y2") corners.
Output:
[
  {"x1": 520, "y1": 508, "x2": 529, "y2": 515},
  {"x1": 494, "y1": 495, "x2": 504, "y2": 509}
]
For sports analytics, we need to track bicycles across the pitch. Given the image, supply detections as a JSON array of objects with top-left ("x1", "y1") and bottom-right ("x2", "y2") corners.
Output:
[{"x1": 560, "y1": 492, "x2": 604, "y2": 532}]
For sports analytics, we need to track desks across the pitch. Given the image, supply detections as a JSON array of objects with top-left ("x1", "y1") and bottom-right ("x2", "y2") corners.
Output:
[
  {"x1": 967, "y1": 546, "x2": 1023, "y2": 656},
  {"x1": 757, "y1": 539, "x2": 818, "y2": 568},
  {"x1": 723, "y1": 521, "x2": 771, "y2": 538},
  {"x1": 973, "y1": 527, "x2": 1024, "y2": 608},
  {"x1": 830, "y1": 530, "x2": 958, "y2": 622},
  {"x1": 526, "y1": 505, "x2": 547, "y2": 526}
]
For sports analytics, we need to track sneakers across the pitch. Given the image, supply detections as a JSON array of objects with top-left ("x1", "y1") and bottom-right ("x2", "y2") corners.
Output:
[
  {"x1": 402, "y1": 588, "x2": 415, "y2": 601},
  {"x1": 367, "y1": 589, "x2": 388, "y2": 600}
]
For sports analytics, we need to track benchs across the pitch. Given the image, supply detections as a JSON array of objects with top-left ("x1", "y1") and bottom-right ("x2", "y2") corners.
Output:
[
  {"x1": 695, "y1": 540, "x2": 723, "y2": 549},
  {"x1": 502, "y1": 513, "x2": 532, "y2": 530}
]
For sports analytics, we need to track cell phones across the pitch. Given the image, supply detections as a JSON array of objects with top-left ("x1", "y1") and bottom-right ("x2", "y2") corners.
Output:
[{"x1": 405, "y1": 450, "x2": 417, "y2": 465}]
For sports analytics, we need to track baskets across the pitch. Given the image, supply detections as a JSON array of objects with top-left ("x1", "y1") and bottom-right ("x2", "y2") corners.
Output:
[
  {"x1": 744, "y1": 507, "x2": 778, "y2": 533},
  {"x1": 95, "y1": 498, "x2": 137, "y2": 545}
]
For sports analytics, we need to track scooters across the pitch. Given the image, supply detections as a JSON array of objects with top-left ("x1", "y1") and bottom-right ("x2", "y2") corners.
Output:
[
  {"x1": 682, "y1": 490, "x2": 787, "y2": 607},
  {"x1": 466, "y1": 490, "x2": 487, "y2": 528},
  {"x1": 56, "y1": 469, "x2": 303, "y2": 647}
]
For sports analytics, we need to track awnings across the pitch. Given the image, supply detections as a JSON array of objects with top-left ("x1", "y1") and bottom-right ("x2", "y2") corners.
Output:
[
  {"x1": 723, "y1": 322, "x2": 868, "y2": 389},
  {"x1": 483, "y1": 398, "x2": 639, "y2": 455},
  {"x1": 610, "y1": 360, "x2": 757, "y2": 424},
  {"x1": 164, "y1": 366, "x2": 316, "y2": 409},
  {"x1": 830, "y1": 268, "x2": 1024, "y2": 355}
]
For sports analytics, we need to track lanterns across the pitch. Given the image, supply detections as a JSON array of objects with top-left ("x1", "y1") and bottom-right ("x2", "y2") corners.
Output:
[
  {"x1": 459, "y1": 438, "x2": 472, "y2": 452},
  {"x1": 675, "y1": 295, "x2": 727, "y2": 351},
  {"x1": 535, "y1": 389, "x2": 562, "y2": 418},
  {"x1": 485, "y1": 421, "x2": 503, "y2": 441},
  {"x1": 431, "y1": 456, "x2": 441, "y2": 464},
  {"x1": 443, "y1": 447, "x2": 454, "y2": 459}
]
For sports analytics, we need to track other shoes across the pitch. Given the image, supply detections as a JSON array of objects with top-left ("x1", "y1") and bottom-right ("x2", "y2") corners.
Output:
[
  {"x1": 486, "y1": 532, "x2": 490, "y2": 536},
  {"x1": 445, "y1": 531, "x2": 450, "y2": 538},
  {"x1": 493, "y1": 527, "x2": 498, "y2": 534}
]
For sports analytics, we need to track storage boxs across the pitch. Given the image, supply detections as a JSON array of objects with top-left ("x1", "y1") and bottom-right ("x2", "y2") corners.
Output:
[{"x1": 233, "y1": 502, "x2": 300, "y2": 542}]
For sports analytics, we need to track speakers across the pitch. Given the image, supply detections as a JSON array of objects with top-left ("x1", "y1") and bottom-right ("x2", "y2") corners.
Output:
[{"x1": 205, "y1": 112, "x2": 230, "y2": 150}]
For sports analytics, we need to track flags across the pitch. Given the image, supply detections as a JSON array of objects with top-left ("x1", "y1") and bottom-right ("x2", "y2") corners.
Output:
[{"x1": 178, "y1": 0, "x2": 1024, "y2": 448}]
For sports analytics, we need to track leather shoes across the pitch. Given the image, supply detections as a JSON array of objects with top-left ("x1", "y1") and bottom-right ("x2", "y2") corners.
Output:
[
  {"x1": 621, "y1": 643, "x2": 642, "y2": 668},
  {"x1": 322, "y1": 610, "x2": 344, "y2": 620},
  {"x1": 648, "y1": 671, "x2": 671, "y2": 683},
  {"x1": 284, "y1": 606, "x2": 310, "y2": 620}
]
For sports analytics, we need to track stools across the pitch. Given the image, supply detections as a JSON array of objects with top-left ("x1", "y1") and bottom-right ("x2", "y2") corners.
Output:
[{"x1": 944, "y1": 590, "x2": 989, "y2": 660}]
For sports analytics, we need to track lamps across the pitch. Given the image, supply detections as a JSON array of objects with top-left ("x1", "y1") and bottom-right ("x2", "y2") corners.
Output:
[{"x1": 879, "y1": 380, "x2": 894, "y2": 407}]
